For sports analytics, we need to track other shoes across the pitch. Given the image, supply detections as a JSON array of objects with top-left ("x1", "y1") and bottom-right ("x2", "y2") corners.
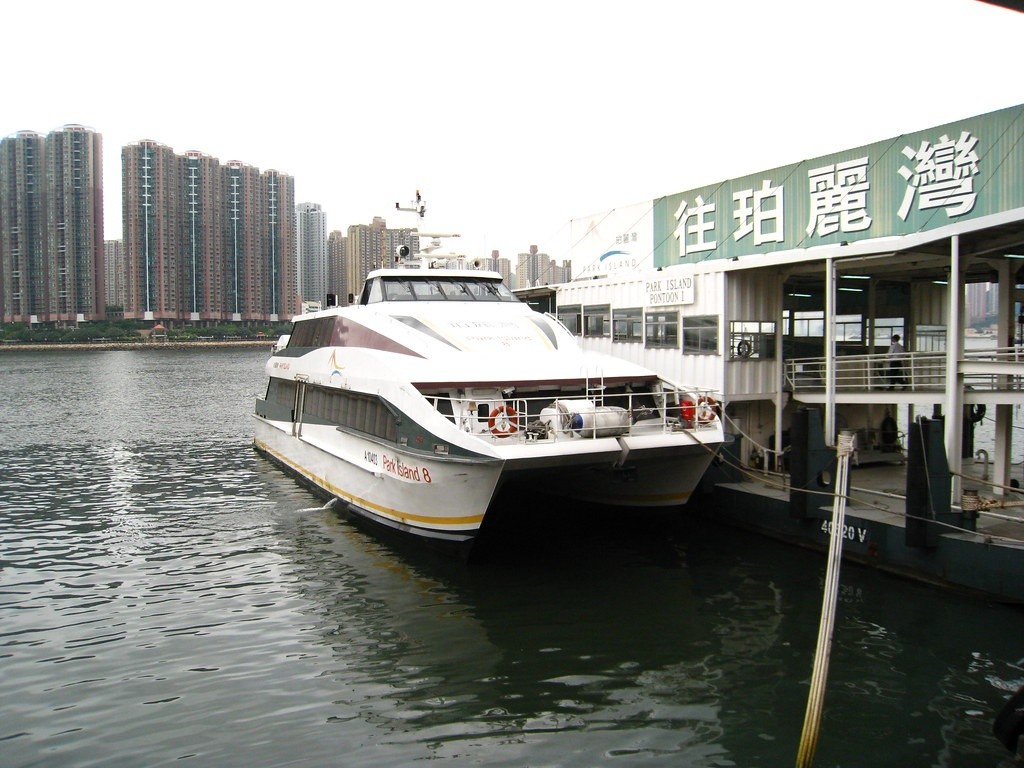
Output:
[
  {"x1": 886, "y1": 388, "x2": 895, "y2": 391},
  {"x1": 901, "y1": 382, "x2": 909, "y2": 391}
]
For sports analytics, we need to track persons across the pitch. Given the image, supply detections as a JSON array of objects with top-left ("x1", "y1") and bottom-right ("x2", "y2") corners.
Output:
[{"x1": 884, "y1": 335, "x2": 908, "y2": 391}]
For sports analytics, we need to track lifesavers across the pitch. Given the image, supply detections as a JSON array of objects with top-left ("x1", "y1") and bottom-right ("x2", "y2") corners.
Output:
[
  {"x1": 967, "y1": 404, "x2": 986, "y2": 424},
  {"x1": 488, "y1": 405, "x2": 518, "y2": 439},
  {"x1": 737, "y1": 340, "x2": 752, "y2": 356},
  {"x1": 694, "y1": 396, "x2": 716, "y2": 425}
]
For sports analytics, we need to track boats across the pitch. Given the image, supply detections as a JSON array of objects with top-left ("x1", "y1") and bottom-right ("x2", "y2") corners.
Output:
[{"x1": 249, "y1": 188, "x2": 737, "y2": 569}]
